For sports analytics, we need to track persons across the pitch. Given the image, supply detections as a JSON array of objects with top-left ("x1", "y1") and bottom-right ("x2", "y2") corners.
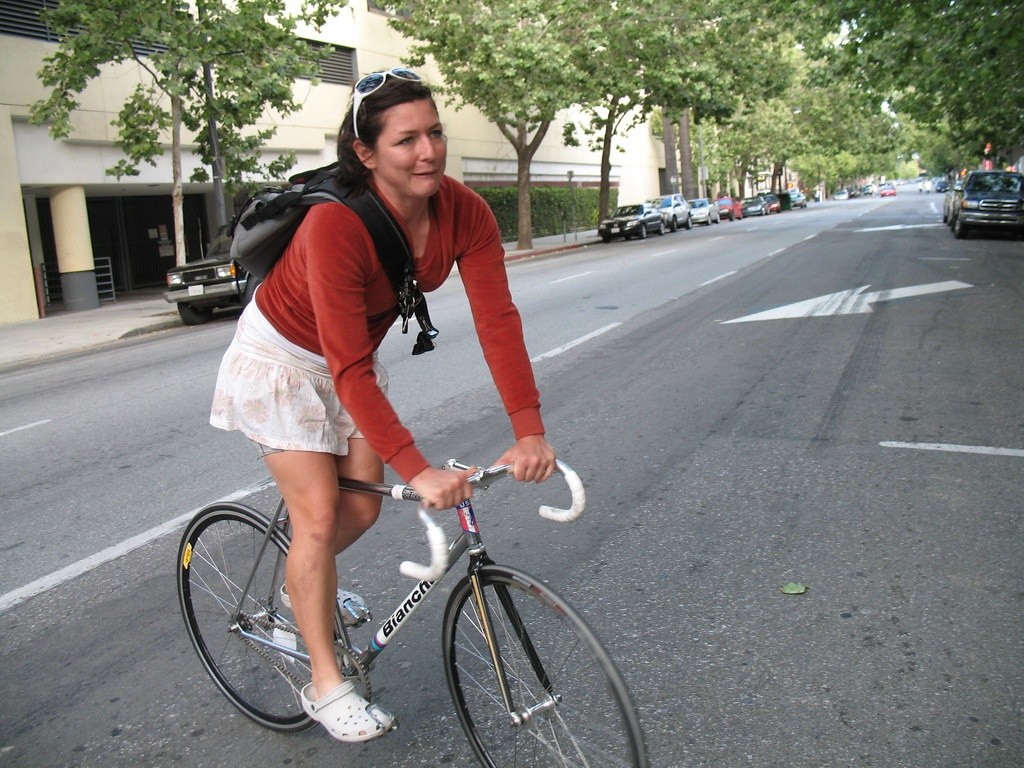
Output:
[{"x1": 208, "y1": 69, "x2": 555, "y2": 743}]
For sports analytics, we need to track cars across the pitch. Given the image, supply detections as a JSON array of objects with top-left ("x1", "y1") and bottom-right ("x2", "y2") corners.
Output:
[
  {"x1": 944, "y1": 180, "x2": 960, "y2": 227},
  {"x1": 598, "y1": 189, "x2": 807, "y2": 244},
  {"x1": 833, "y1": 177, "x2": 950, "y2": 200}
]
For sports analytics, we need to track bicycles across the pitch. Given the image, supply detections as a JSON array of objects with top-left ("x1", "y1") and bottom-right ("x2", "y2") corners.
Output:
[{"x1": 172, "y1": 453, "x2": 651, "y2": 767}]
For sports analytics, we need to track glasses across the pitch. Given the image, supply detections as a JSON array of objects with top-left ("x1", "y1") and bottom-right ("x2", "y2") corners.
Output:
[{"x1": 354, "y1": 68, "x2": 422, "y2": 139}]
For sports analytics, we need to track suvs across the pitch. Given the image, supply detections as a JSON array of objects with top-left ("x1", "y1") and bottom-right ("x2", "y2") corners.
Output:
[
  {"x1": 955, "y1": 171, "x2": 1024, "y2": 243},
  {"x1": 162, "y1": 222, "x2": 264, "y2": 325}
]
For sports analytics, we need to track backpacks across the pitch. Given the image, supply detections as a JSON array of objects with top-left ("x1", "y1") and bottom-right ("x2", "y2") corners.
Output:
[{"x1": 230, "y1": 166, "x2": 421, "y2": 335}]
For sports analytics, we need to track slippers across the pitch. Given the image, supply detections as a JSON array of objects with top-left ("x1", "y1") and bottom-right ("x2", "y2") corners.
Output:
[
  {"x1": 300, "y1": 677, "x2": 394, "y2": 743},
  {"x1": 280, "y1": 578, "x2": 367, "y2": 627}
]
